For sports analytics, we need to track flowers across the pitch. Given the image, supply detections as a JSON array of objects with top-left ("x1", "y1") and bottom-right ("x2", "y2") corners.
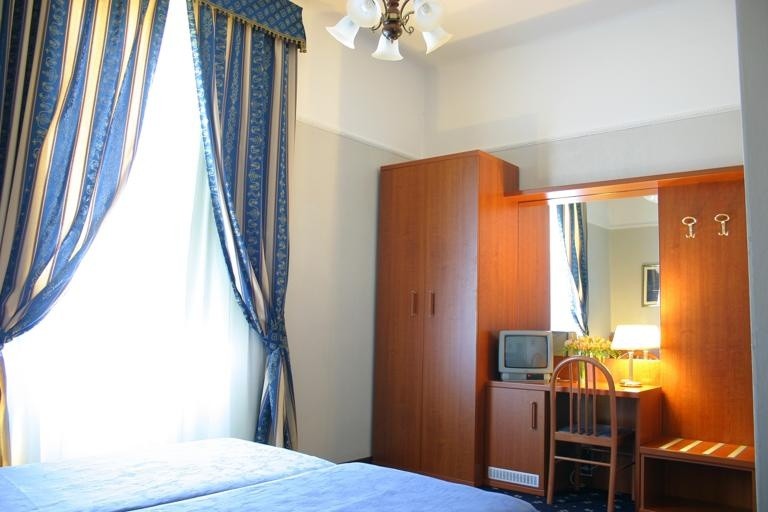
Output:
[{"x1": 564, "y1": 337, "x2": 616, "y2": 359}]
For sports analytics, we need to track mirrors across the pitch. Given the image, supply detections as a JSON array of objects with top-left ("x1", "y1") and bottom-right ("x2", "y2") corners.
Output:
[{"x1": 519, "y1": 187, "x2": 660, "y2": 363}]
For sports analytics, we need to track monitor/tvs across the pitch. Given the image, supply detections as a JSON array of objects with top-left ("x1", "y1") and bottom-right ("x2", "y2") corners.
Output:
[
  {"x1": 552, "y1": 331, "x2": 578, "y2": 357},
  {"x1": 498, "y1": 330, "x2": 553, "y2": 383}
]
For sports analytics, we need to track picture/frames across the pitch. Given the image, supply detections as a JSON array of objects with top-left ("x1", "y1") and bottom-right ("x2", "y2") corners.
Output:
[{"x1": 641, "y1": 264, "x2": 659, "y2": 307}]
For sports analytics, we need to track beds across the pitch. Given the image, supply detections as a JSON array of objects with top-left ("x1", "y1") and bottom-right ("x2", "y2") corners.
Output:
[{"x1": 0, "y1": 435, "x2": 539, "y2": 512}]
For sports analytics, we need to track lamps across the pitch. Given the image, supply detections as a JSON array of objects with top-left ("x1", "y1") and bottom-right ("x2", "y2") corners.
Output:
[
  {"x1": 612, "y1": 324, "x2": 647, "y2": 387},
  {"x1": 643, "y1": 325, "x2": 660, "y2": 360},
  {"x1": 324, "y1": 0, "x2": 453, "y2": 62}
]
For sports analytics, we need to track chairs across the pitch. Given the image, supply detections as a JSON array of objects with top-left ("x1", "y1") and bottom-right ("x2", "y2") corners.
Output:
[{"x1": 545, "y1": 358, "x2": 633, "y2": 510}]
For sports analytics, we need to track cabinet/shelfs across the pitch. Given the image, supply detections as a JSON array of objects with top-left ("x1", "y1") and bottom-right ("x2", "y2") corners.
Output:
[
  {"x1": 370, "y1": 149, "x2": 519, "y2": 488},
  {"x1": 639, "y1": 437, "x2": 757, "y2": 511},
  {"x1": 520, "y1": 204, "x2": 551, "y2": 330},
  {"x1": 483, "y1": 381, "x2": 661, "y2": 512}
]
